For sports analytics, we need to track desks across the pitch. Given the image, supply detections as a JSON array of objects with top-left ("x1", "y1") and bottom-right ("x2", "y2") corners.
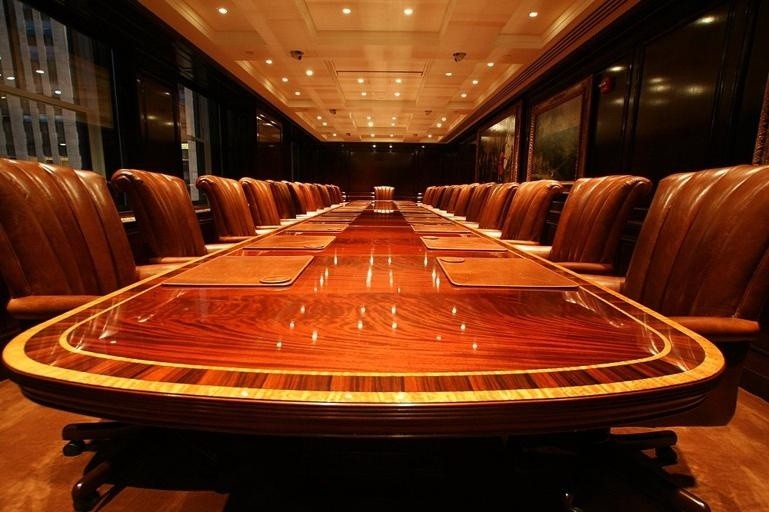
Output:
[{"x1": 0, "y1": 198, "x2": 729, "y2": 512}]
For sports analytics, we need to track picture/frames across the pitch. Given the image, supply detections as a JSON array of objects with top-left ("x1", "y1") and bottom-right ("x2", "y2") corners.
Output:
[
  {"x1": 254, "y1": 108, "x2": 283, "y2": 182},
  {"x1": 474, "y1": 100, "x2": 523, "y2": 184},
  {"x1": 525, "y1": 75, "x2": 594, "y2": 196}
]
